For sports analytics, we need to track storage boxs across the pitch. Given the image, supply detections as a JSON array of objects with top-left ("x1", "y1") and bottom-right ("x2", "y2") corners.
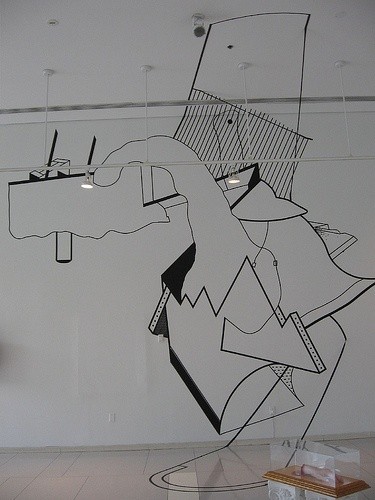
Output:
[{"x1": 262, "y1": 464, "x2": 372, "y2": 500}]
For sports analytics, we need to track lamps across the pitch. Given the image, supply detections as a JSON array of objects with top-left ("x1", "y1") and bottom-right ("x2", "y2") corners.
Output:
[
  {"x1": 78, "y1": 166, "x2": 98, "y2": 188},
  {"x1": 226, "y1": 163, "x2": 240, "y2": 184}
]
